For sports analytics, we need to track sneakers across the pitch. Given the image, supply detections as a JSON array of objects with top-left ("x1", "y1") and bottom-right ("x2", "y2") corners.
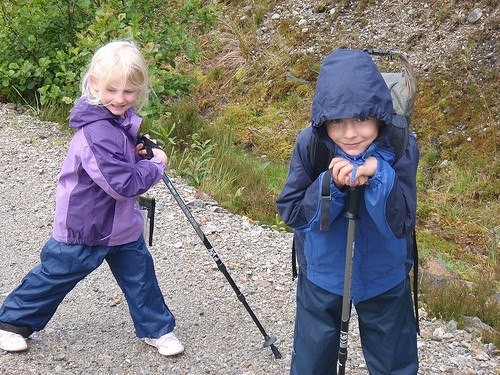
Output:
[
  {"x1": 145, "y1": 332, "x2": 184, "y2": 356},
  {"x1": 0, "y1": 329, "x2": 27, "y2": 351}
]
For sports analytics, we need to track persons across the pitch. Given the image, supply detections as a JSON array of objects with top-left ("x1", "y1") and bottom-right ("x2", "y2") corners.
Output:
[
  {"x1": 0, "y1": 40, "x2": 185, "y2": 356},
  {"x1": 276, "y1": 49, "x2": 420, "y2": 375}
]
